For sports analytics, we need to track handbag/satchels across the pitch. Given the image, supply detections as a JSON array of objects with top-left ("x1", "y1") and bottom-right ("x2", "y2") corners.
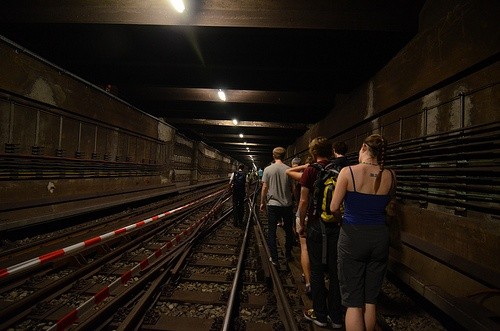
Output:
[{"x1": 385, "y1": 169, "x2": 400, "y2": 241}]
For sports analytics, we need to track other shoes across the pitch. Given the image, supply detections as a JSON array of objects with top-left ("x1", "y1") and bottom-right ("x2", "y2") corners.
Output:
[
  {"x1": 305, "y1": 284, "x2": 311, "y2": 294},
  {"x1": 302, "y1": 273, "x2": 305, "y2": 280},
  {"x1": 269, "y1": 257, "x2": 277, "y2": 265}
]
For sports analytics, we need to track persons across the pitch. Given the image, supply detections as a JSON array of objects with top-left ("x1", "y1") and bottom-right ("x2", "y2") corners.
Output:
[
  {"x1": 291, "y1": 157, "x2": 301, "y2": 247},
  {"x1": 286, "y1": 157, "x2": 314, "y2": 292},
  {"x1": 299, "y1": 136, "x2": 344, "y2": 328},
  {"x1": 259, "y1": 147, "x2": 296, "y2": 265},
  {"x1": 331, "y1": 141, "x2": 349, "y2": 172},
  {"x1": 330, "y1": 133, "x2": 396, "y2": 331},
  {"x1": 247, "y1": 168, "x2": 263, "y2": 185},
  {"x1": 229, "y1": 164, "x2": 247, "y2": 227}
]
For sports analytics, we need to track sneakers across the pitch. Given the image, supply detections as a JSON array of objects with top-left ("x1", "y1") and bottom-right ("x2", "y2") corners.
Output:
[
  {"x1": 327, "y1": 315, "x2": 342, "y2": 328},
  {"x1": 303, "y1": 307, "x2": 327, "y2": 326}
]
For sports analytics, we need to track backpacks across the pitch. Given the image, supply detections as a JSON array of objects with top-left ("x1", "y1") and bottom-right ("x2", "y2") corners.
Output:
[
  {"x1": 309, "y1": 160, "x2": 345, "y2": 223},
  {"x1": 233, "y1": 171, "x2": 246, "y2": 191}
]
